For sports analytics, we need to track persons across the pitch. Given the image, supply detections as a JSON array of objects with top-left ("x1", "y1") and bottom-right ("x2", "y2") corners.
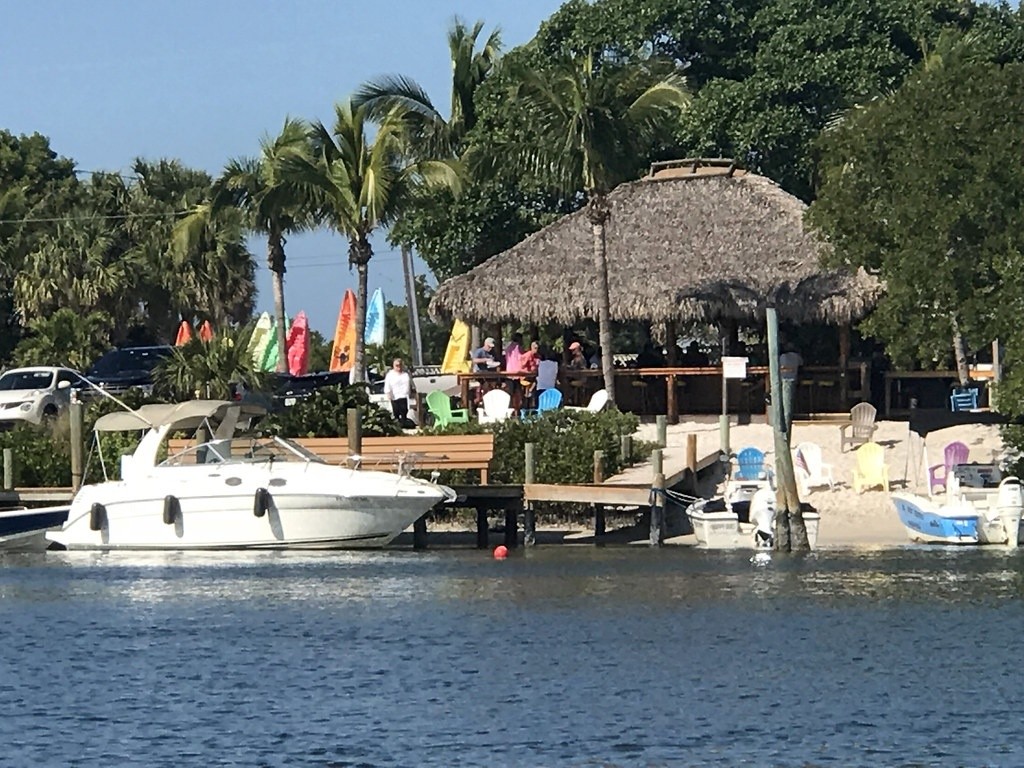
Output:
[
  {"x1": 637, "y1": 339, "x2": 950, "y2": 427},
  {"x1": 475, "y1": 333, "x2": 587, "y2": 405},
  {"x1": 384, "y1": 356, "x2": 412, "y2": 419}
]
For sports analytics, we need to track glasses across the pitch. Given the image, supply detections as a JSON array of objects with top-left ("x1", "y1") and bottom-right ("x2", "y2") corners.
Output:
[{"x1": 534, "y1": 347, "x2": 539, "y2": 349}]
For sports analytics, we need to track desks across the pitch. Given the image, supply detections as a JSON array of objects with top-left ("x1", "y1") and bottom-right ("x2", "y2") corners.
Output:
[
  {"x1": 459, "y1": 373, "x2": 537, "y2": 418},
  {"x1": 955, "y1": 463, "x2": 1002, "y2": 488}
]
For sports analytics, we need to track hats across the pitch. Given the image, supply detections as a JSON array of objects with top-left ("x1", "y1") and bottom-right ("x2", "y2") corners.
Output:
[
  {"x1": 511, "y1": 332, "x2": 523, "y2": 342},
  {"x1": 531, "y1": 342, "x2": 539, "y2": 348},
  {"x1": 485, "y1": 337, "x2": 495, "y2": 347},
  {"x1": 568, "y1": 342, "x2": 580, "y2": 350}
]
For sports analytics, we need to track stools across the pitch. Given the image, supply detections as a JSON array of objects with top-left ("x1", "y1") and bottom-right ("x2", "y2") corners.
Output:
[
  {"x1": 569, "y1": 381, "x2": 585, "y2": 407},
  {"x1": 630, "y1": 380, "x2": 648, "y2": 415},
  {"x1": 795, "y1": 380, "x2": 815, "y2": 413},
  {"x1": 678, "y1": 381, "x2": 686, "y2": 388},
  {"x1": 816, "y1": 380, "x2": 836, "y2": 413}
]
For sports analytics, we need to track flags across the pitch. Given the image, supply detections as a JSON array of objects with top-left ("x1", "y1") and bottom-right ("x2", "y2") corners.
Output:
[{"x1": 795, "y1": 450, "x2": 811, "y2": 475}]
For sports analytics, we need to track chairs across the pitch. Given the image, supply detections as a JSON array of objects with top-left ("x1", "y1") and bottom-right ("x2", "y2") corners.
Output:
[
  {"x1": 520, "y1": 388, "x2": 562, "y2": 423},
  {"x1": 985, "y1": 476, "x2": 1024, "y2": 525},
  {"x1": 847, "y1": 362, "x2": 872, "y2": 404},
  {"x1": 849, "y1": 442, "x2": 891, "y2": 496},
  {"x1": 564, "y1": 388, "x2": 608, "y2": 416},
  {"x1": 426, "y1": 389, "x2": 470, "y2": 432},
  {"x1": 929, "y1": 441, "x2": 970, "y2": 494},
  {"x1": 734, "y1": 447, "x2": 777, "y2": 491},
  {"x1": 794, "y1": 441, "x2": 835, "y2": 496},
  {"x1": 476, "y1": 389, "x2": 516, "y2": 424},
  {"x1": 839, "y1": 401, "x2": 878, "y2": 453}
]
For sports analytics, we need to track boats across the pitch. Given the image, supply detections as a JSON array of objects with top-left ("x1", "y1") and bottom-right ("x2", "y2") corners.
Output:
[
  {"x1": 684, "y1": 480, "x2": 822, "y2": 552},
  {"x1": 888, "y1": 474, "x2": 1024, "y2": 548},
  {"x1": 42, "y1": 361, "x2": 459, "y2": 554}
]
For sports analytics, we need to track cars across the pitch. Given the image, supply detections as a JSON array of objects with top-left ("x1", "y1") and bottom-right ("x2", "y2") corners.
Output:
[
  {"x1": 218, "y1": 370, "x2": 389, "y2": 429},
  {"x1": 0, "y1": 365, "x2": 84, "y2": 432},
  {"x1": 74, "y1": 343, "x2": 178, "y2": 399},
  {"x1": 364, "y1": 377, "x2": 419, "y2": 431}
]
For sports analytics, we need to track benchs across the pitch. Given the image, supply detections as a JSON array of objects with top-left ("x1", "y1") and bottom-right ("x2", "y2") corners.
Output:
[
  {"x1": 201, "y1": 439, "x2": 251, "y2": 463},
  {"x1": 362, "y1": 433, "x2": 495, "y2": 486},
  {"x1": 255, "y1": 437, "x2": 349, "y2": 467}
]
